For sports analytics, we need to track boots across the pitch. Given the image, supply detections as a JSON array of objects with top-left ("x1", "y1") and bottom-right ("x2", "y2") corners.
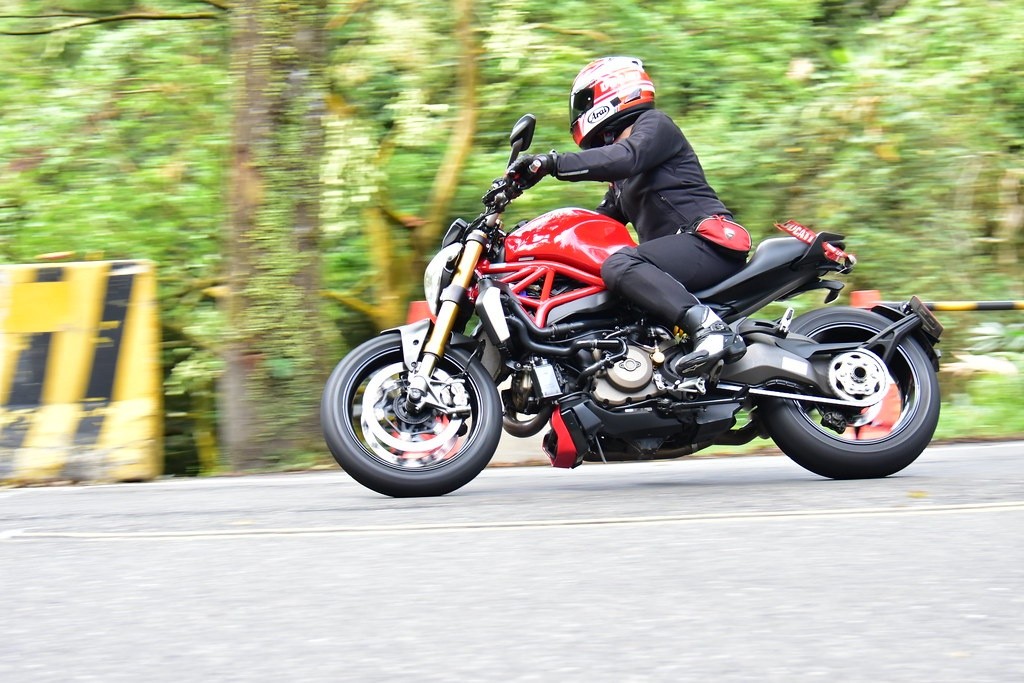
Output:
[{"x1": 675, "y1": 304, "x2": 747, "y2": 378}]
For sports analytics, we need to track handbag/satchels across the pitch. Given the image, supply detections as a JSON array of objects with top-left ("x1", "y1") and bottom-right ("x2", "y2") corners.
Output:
[{"x1": 692, "y1": 215, "x2": 752, "y2": 257}]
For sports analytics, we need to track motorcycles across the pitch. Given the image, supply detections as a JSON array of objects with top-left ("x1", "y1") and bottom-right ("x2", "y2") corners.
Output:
[{"x1": 320, "y1": 115, "x2": 947, "y2": 499}]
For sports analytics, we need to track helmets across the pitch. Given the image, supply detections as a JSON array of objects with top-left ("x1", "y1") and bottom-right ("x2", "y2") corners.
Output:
[{"x1": 570, "y1": 57, "x2": 654, "y2": 148}]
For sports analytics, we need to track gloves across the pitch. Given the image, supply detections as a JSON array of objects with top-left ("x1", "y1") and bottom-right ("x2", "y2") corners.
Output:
[{"x1": 505, "y1": 154, "x2": 554, "y2": 189}]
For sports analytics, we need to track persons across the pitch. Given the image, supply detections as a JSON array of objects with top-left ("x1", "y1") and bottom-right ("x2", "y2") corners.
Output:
[{"x1": 504, "y1": 55, "x2": 751, "y2": 379}]
